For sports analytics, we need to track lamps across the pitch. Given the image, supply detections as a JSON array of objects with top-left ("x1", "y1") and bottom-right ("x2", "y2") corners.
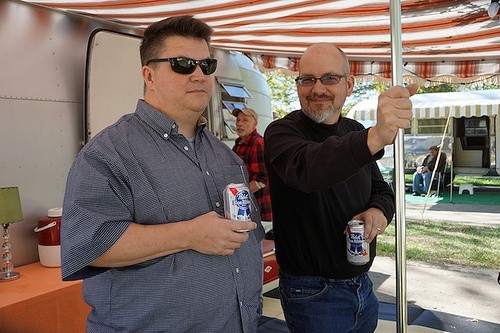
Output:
[{"x1": 0, "y1": 186, "x2": 24, "y2": 281}]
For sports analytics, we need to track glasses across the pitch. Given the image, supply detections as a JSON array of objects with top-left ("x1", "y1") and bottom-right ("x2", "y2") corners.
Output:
[
  {"x1": 146, "y1": 57, "x2": 217, "y2": 75},
  {"x1": 295, "y1": 74, "x2": 347, "y2": 87}
]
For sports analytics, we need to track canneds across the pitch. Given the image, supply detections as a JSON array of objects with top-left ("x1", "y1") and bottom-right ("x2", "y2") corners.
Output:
[
  {"x1": 223, "y1": 182, "x2": 252, "y2": 233},
  {"x1": 347, "y1": 219, "x2": 370, "y2": 266}
]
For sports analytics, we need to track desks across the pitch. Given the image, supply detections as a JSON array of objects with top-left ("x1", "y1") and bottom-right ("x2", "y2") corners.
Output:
[{"x1": 0, "y1": 263, "x2": 94, "y2": 333}]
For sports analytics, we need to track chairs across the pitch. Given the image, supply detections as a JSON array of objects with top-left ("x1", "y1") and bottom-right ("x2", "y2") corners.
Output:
[{"x1": 413, "y1": 155, "x2": 447, "y2": 198}]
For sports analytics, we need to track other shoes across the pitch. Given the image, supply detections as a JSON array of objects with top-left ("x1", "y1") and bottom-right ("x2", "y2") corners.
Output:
[
  {"x1": 412, "y1": 192, "x2": 419, "y2": 196},
  {"x1": 421, "y1": 193, "x2": 426, "y2": 196}
]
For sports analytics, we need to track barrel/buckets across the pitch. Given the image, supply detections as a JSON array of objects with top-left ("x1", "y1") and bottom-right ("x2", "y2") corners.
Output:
[{"x1": 34, "y1": 207, "x2": 61, "y2": 268}]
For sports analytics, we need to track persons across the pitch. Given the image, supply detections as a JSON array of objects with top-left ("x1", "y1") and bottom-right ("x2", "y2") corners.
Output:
[
  {"x1": 231, "y1": 108, "x2": 272, "y2": 222},
  {"x1": 412, "y1": 146, "x2": 446, "y2": 196},
  {"x1": 60, "y1": 16, "x2": 265, "y2": 333},
  {"x1": 264, "y1": 43, "x2": 419, "y2": 333}
]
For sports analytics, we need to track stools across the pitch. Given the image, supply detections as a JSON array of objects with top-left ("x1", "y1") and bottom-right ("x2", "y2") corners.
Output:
[{"x1": 458, "y1": 183, "x2": 474, "y2": 195}]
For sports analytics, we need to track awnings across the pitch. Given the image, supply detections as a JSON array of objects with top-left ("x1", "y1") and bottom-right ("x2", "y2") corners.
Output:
[{"x1": 354, "y1": 89, "x2": 500, "y2": 121}]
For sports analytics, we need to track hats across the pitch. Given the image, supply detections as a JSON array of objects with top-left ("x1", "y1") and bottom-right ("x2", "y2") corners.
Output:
[
  {"x1": 430, "y1": 146, "x2": 438, "y2": 151},
  {"x1": 232, "y1": 108, "x2": 258, "y2": 125}
]
